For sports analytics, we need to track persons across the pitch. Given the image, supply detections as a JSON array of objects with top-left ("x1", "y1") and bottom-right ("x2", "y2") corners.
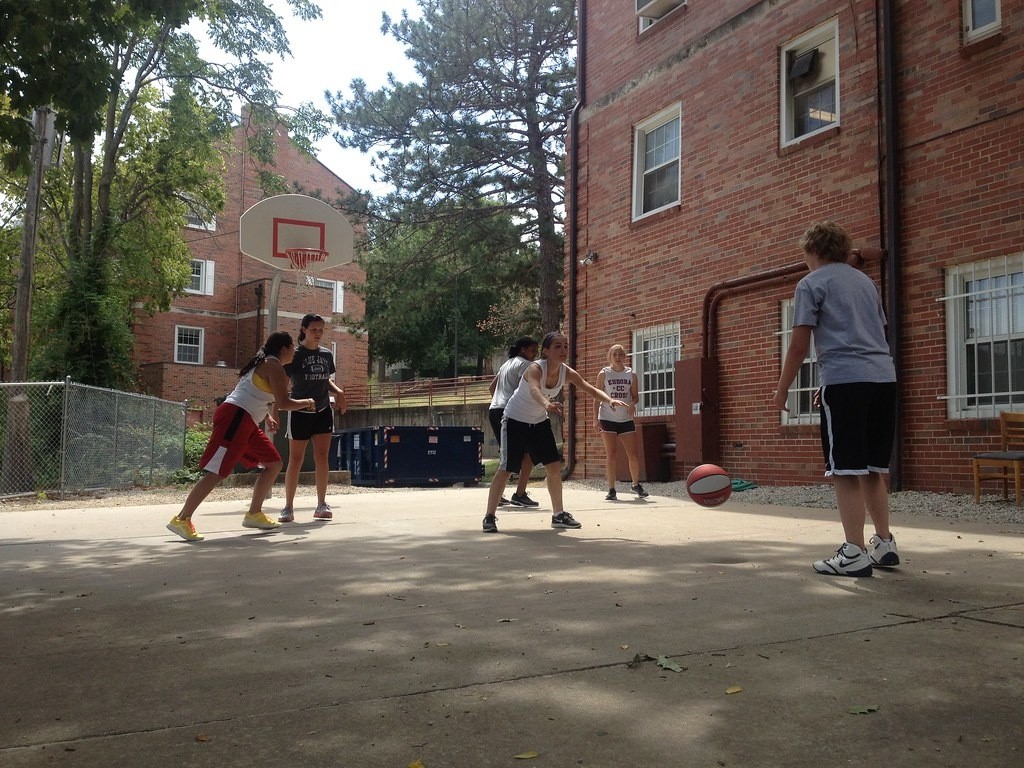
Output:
[
  {"x1": 775, "y1": 219, "x2": 900, "y2": 577},
  {"x1": 594, "y1": 344, "x2": 649, "y2": 501},
  {"x1": 165, "y1": 331, "x2": 316, "y2": 541},
  {"x1": 273, "y1": 313, "x2": 347, "y2": 523},
  {"x1": 482, "y1": 333, "x2": 630, "y2": 532},
  {"x1": 488, "y1": 337, "x2": 540, "y2": 508}
]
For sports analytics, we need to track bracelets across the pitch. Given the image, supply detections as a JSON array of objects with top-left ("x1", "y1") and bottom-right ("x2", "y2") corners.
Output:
[{"x1": 308, "y1": 398, "x2": 312, "y2": 410}]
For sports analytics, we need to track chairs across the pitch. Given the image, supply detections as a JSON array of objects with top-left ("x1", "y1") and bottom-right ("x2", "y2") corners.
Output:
[{"x1": 972, "y1": 411, "x2": 1024, "y2": 507}]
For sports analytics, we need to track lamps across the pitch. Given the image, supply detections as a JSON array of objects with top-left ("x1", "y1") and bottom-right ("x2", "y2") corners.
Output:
[{"x1": 580, "y1": 252, "x2": 597, "y2": 267}]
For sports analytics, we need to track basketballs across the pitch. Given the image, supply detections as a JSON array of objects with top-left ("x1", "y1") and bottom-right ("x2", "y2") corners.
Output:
[{"x1": 685, "y1": 463, "x2": 732, "y2": 509}]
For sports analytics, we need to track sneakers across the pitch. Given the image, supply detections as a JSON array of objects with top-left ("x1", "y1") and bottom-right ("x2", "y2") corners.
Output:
[
  {"x1": 313, "y1": 502, "x2": 332, "y2": 518},
  {"x1": 606, "y1": 488, "x2": 617, "y2": 500},
  {"x1": 278, "y1": 506, "x2": 294, "y2": 521},
  {"x1": 552, "y1": 511, "x2": 581, "y2": 528},
  {"x1": 483, "y1": 514, "x2": 498, "y2": 532},
  {"x1": 868, "y1": 532, "x2": 900, "y2": 566},
  {"x1": 630, "y1": 483, "x2": 649, "y2": 498},
  {"x1": 242, "y1": 511, "x2": 280, "y2": 529},
  {"x1": 166, "y1": 516, "x2": 204, "y2": 541},
  {"x1": 510, "y1": 492, "x2": 539, "y2": 507},
  {"x1": 500, "y1": 494, "x2": 510, "y2": 505},
  {"x1": 813, "y1": 542, "x2": 873, "y2": 577}
]
